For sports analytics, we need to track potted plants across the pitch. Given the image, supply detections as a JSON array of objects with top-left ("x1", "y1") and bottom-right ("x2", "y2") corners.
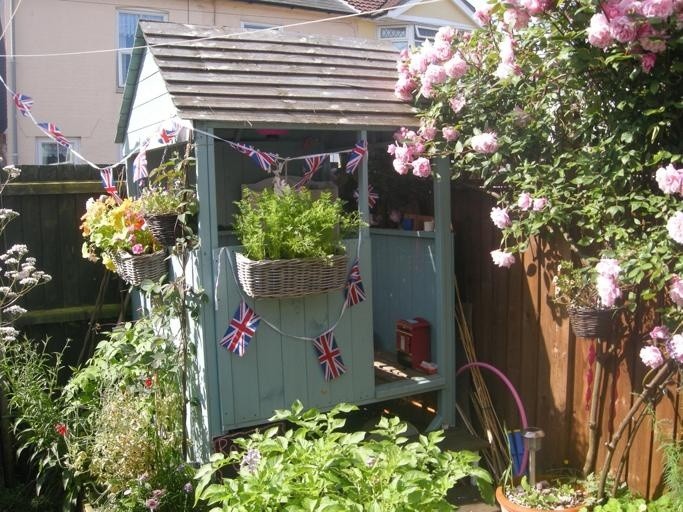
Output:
[
  {"x1": 137, "y1": 160, "x2": 192, "y2": 246},
  {"x1": 227, "y1": 176, "x2": 364, "y2": 303}
]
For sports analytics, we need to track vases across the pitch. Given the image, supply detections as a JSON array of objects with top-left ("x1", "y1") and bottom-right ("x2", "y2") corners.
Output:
[{"x1": 496, "y1": 472, "x2": 590, "y2": 512}]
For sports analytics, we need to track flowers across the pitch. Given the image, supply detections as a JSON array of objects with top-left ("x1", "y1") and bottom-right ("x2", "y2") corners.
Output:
[{"x1": 73, "y1": 193, "x2": 153, "y2": 273}]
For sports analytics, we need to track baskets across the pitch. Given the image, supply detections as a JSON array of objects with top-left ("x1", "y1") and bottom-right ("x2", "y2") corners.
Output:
[
  {"x1": 142, "y1": 214, "x2": 180, "y2": 245},
  {"x1": 105, "y1": 247, "x2": 166, "y2": 287},
  {"x1": 235, "y1": 244, "x2": 348, "y2": 300},
  {"x1": 566, "y1": 304, "x2": 613, "y2": 339}
]
